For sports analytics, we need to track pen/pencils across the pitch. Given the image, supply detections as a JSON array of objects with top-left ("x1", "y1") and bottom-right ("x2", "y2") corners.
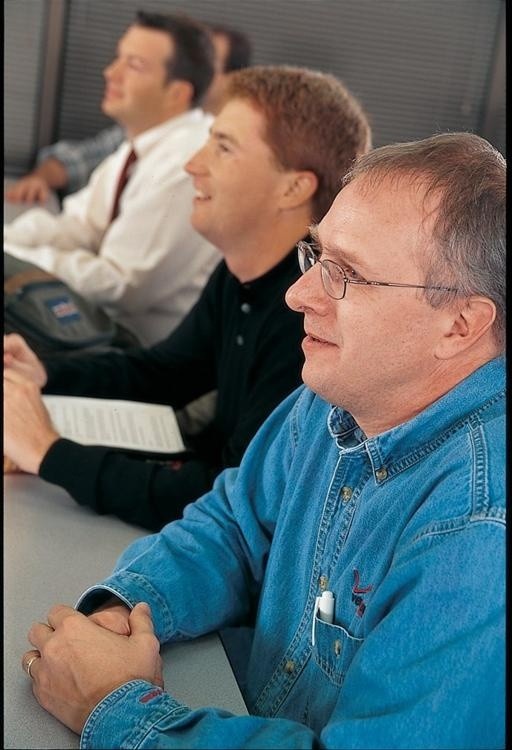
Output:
[{"x1": 311, "y1": 590, "x2": 335, "y2": 647}]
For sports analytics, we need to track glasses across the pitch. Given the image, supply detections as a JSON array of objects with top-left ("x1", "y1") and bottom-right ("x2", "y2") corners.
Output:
[{"x1": 294, "y1": 239, "x2": 459, "y2": 300}]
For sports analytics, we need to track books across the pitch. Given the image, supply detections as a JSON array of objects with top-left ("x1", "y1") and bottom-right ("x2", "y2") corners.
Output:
[{"x1": 42, "y1": 393, "x2": 188, "y2": 454}]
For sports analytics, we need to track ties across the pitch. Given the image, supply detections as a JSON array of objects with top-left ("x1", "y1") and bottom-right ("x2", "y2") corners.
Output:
[{"x1": 107, "y1": 149, "x2": 137, "y2": 222}]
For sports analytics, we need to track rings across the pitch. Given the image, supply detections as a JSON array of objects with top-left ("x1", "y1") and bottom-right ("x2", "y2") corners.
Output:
[{"x1": 22, "y1": 654, "x2": 39, "y2": 679}]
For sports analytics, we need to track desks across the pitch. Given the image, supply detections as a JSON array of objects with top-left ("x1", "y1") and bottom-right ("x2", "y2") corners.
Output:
[{"x1": 3, "y1": 175, "x2": 251, "y2": 749}]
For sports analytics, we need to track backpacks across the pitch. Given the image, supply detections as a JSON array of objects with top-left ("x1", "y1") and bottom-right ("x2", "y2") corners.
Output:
[{"x1": 4, "y1": 252, "x2": 142, "y2": 360}]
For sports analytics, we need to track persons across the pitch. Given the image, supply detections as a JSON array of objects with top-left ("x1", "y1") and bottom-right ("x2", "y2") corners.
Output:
[
  {"x1": 0, "y1": 69, "x2": 374, "y2": 528},
  {"x1": 3, "y1": 24, "x2": 248, "y2": 208},
  {"x1": 0, "y1": 9, "x2": 221, "y2": 438},
  {"x1": 1, "y1": 131, "x2": 506, "y2": 750}
]
